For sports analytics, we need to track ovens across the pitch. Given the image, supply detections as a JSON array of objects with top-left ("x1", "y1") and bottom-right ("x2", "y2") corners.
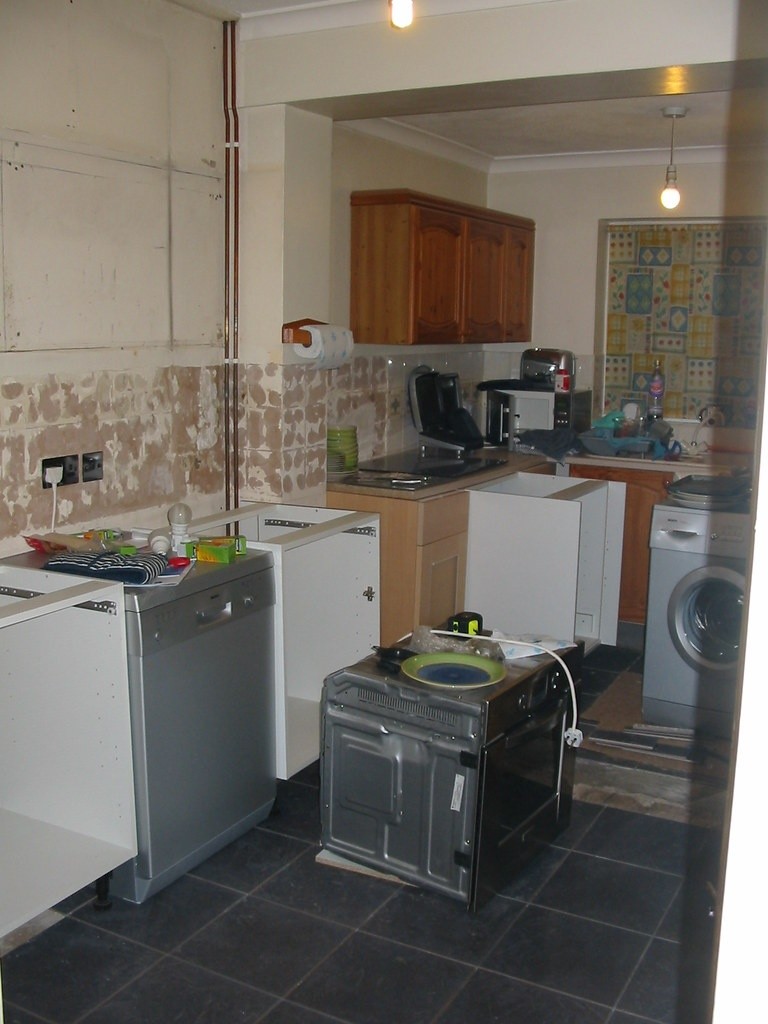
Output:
[{"x1": 319, "y1": 622, "x2": 585, "y2": 911}]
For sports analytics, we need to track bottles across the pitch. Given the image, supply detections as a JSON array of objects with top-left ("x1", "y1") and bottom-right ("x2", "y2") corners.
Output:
[
  {"x1": 647, "y1": 360, "x2": 665, "y2": 419},
  {"x1": 68, "y1": 527, "x2": 131, "y2": 542}
]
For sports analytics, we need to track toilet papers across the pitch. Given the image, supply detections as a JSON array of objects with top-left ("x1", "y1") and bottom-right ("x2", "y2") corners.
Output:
[{"x1": 294, "y1": 324, "x2": 354, "y2": 369}]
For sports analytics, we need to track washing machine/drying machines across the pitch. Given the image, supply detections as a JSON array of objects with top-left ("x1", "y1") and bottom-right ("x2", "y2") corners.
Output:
[{"x1": 640, "y1": 473, "x2": 757, "y2": 733}]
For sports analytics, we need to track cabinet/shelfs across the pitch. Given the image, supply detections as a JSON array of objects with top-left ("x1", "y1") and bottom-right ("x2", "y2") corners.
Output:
[
  {"x1": 327, "y1": 447, "x2": 556, "y2": 648},
  {"x1": 503, "y1": 214, "x2": 534, "y2": 342},
  {"x1": 462, "y1": 204, "x2": 503, "y2": 343},
  {"x1": 569, "y1": 464, "x2": 675, "y2": 624},
  {"x1": 350, "y1": 190, "x2": 464, "y2": 346}
]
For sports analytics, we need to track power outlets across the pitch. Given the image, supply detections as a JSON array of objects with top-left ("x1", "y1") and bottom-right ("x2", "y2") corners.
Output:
[
  {"x1": 82, "y1": 451, "x2": 103, "y2": 482},
  {"x1": 42, "y1": 454, "x2": 79, "y2": 488}
]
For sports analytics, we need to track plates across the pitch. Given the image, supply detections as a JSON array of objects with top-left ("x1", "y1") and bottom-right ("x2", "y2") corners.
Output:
[
  {"x1": 667, "y1": 475, "x2": 752, "y2": 510},
  {"x1": 402, "y1": 653, "x2": 507, "y2": 689},
  {"x1": 326, "y1": 426, "x2": 359, "y2": 481}
]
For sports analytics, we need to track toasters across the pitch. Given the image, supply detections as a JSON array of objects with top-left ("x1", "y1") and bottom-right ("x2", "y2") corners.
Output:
[{"x1": 519, "y1": 347, "x2": 575, "y2": 392}]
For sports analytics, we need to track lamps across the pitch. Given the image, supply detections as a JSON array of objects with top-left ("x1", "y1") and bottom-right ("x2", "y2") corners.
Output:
[{"x1": 658, "y1": 106, "x2": 691, "y2": 211}]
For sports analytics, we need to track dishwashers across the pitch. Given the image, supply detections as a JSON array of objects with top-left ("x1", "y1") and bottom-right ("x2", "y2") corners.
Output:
[{"x1": 1, "y1": 532, "x2": 279, "y2": 904}]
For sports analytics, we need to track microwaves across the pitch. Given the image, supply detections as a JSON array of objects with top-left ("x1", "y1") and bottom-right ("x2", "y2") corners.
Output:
[{"x1": 476, "y1": 379, "x2": 592, "y2": 441}]
